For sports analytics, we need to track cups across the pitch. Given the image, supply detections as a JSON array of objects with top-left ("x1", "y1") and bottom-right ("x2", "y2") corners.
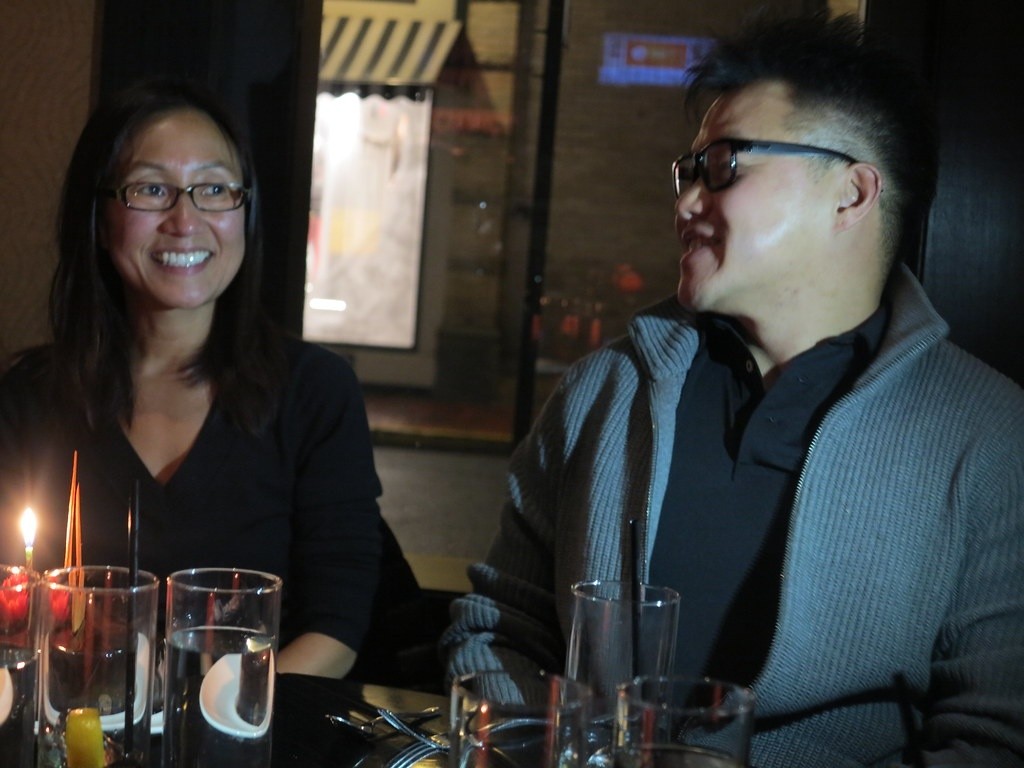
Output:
[
  {"x1": 448, "y1": 669, "x2": 595, "y2": 768},
  {"x1": 35, "y1": 565, "x2": 161, "y2": 768},
  {"x1": 161, "y1": 567, "x2": 283, "y2": 768},
  {"x1": 0, "y1": 562, "x2": 43, "y2": 767},
  {"x1": 607, "y1": 677, "x2": 757, "y2": 768},
  {"x1": 554, "y1": 579, "x2": 681, "y2": 768}
]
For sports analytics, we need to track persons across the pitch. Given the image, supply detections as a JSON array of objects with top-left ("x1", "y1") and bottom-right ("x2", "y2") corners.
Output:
[
  {"x1": 436, "y1": 0, "x2": 1024, "y2": 767},
  {"x1": 0, "y1": 93, "x2": 387, "y2": 684}
]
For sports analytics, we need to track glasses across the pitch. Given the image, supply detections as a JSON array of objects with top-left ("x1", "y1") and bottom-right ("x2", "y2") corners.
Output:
[
  {"x1": 671, "y1": 137, "x2": 884, "y2": 200},
  {"x1": 106, "y1": 181, "x2": 250, "y2": 212}
]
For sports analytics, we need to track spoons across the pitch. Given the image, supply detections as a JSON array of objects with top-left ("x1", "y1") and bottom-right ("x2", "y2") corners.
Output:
[{"x1": 324, "y1": 706, "x2": 441, "y2": 738}]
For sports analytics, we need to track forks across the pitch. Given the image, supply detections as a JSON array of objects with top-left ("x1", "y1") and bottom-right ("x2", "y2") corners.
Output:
[
  {"x1": 380, "y1": 716, "x2": 614, "y2": 768},
  {"x1": 376, "y1": 708, "x2": 547, "y2": 753}
]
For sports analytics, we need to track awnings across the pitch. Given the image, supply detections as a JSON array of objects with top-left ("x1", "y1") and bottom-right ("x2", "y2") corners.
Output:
[{"x1": 318, "y1": 14, "x2": 461, "y2": 102}]
